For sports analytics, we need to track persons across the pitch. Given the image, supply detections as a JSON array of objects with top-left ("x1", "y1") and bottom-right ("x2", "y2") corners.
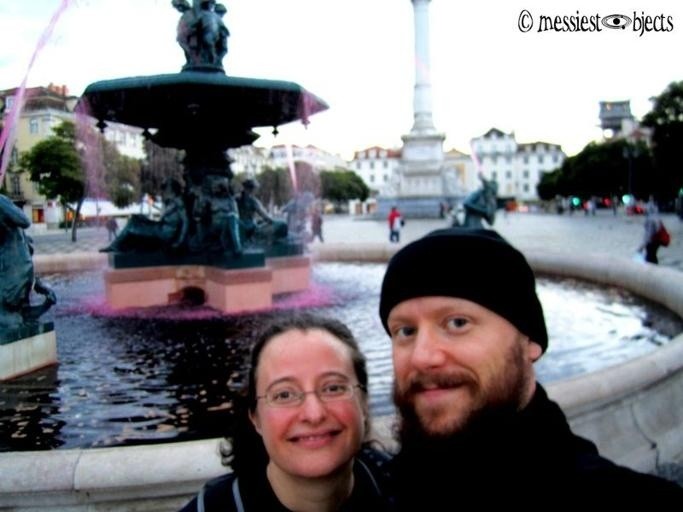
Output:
[
  {"x1": 376, "y1": 225, "x2": 682, "y2": 510},
  {"x1": 448, "y1": 173, "x2": 497, "y2": 229},
  {"x1": 97, "y1": 177, "x2": 188, "y2": 254},
  {"x1": 387, "y1": 206, "x2": 401, "y2": 241},
  {"x1": 238, "y1": 176, "x2": 289, "y2": 238},
  {"x1": 187, "y1": 174, "x2": 246, "y2": 258},
  {"x1": 178, "y1": 311, "x2": 398, "y2": 511},
  {"x1": 633, "y1": 202, "x2": 660, "y2": 264},
  {"x1": 105, "y1": 216, "x2": 118, "y2": 241},
  {"x1": 308, "y1": 207, "x2": 324, "y2": 243}
]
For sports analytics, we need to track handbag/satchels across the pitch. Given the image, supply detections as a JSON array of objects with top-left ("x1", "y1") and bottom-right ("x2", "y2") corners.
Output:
[{"x1": 652, "y1": 223, "x2": 668, "y2": 245}]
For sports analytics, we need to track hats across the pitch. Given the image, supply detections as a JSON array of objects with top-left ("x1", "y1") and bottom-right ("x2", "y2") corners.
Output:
[{"x1": 380, "y1": 227, "x2": 545, "y2": 353}]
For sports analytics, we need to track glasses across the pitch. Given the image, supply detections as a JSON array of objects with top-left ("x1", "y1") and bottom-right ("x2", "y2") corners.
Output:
[{"x1": 253, "y1": 383, "x2": 362, "y2": 406}]
